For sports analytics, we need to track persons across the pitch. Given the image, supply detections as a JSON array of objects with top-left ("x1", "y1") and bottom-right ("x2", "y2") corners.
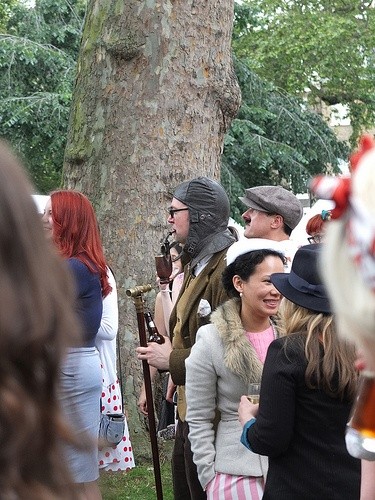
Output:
[
  {"x1": 41, "y1": 189, "x2": 112, "y2": 500},
  {"x1": 0, "y1": 137, "x2": 106, "y2": 500},
  {"x1": 96, "y1": 135, "x2": 375, "y2": 500}
]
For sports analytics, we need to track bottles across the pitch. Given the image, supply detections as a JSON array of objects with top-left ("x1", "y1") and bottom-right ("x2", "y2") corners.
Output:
[{"x1": 144, "y1": 312, "x2": 167, "y2": 373}]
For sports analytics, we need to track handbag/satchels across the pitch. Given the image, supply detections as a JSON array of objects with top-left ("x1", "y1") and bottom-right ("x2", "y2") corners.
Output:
[{"x1": 98, "y1": 413, "x2": 125, "y2": 449}]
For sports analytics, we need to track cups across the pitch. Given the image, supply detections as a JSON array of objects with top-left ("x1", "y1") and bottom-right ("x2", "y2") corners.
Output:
[{"x1": 247, "y1": 383, "x2": 260, "y2": 404}]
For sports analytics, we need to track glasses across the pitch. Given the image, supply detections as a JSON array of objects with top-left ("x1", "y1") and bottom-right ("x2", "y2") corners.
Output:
[
  {"x1": 308, "y1": 234, "x2": 323, "y2": 245},
  {"x1": 168, "y1": 208, "x2": 189, "y2": 218}
]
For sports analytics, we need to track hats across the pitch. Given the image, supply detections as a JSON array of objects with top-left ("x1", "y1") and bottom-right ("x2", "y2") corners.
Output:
[
  {"x1": 238, "y1": 185, "x2": 303, "y2": 230},
  {"x1": 226, "y1": 238, "x2": 290, "y2": 267},
  {"x1": 270, "y1": 243, "x2": 331, "y2": 315},
  {"x1": 167, "y1": 177, "x2": 237, "y2": 268}
]
